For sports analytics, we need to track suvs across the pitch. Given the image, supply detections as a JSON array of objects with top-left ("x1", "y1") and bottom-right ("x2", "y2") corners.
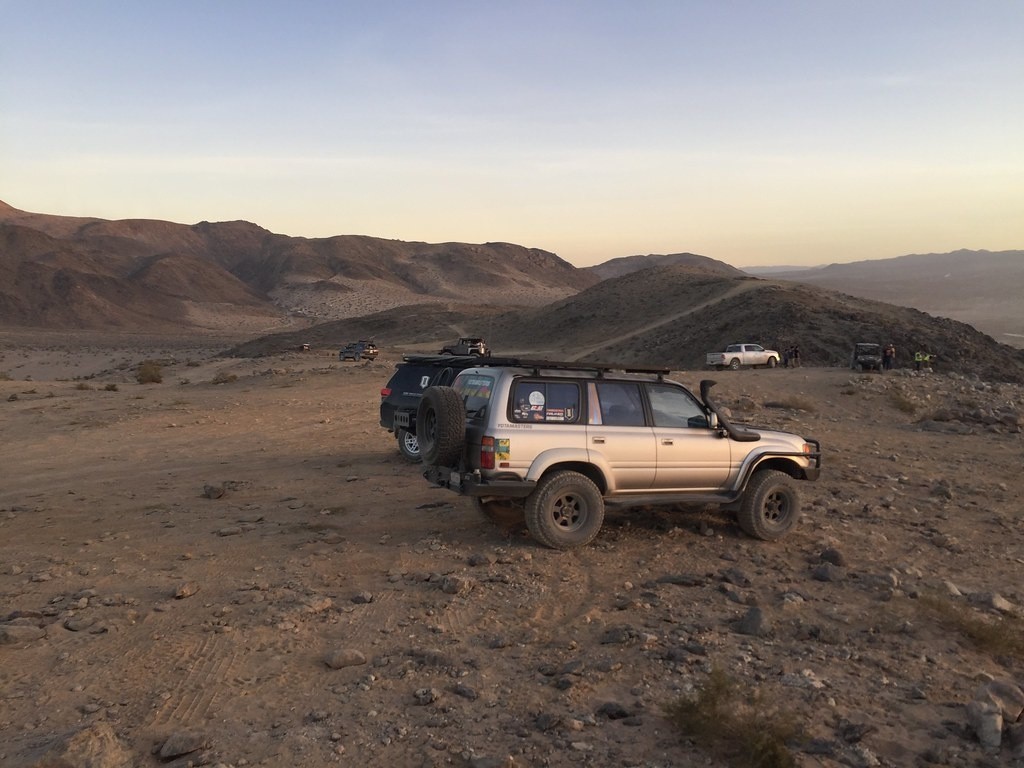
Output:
[
  {"x1": 414, "y1": 354, "x2": 822, "y2": 542},
  {"x1": 850, "y1": 343, "x2": 884, "y2": 375},
  {"x1": 437, "y1": 338, "x2": 491, "y2": 358},
  {"x1": 338, "y1": 340, "x2": 379, "y2": 362},
  {"x1": 378, "y1": 351, "x2": 642, "y2": 463}
]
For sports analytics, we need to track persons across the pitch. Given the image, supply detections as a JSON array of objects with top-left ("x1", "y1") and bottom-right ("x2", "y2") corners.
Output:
[
  {"x1": 784, "y1": 346, "x2": 801, "y2": 368},
  {"x1": 914, "y1": 349, "x2": 936, "y2": 372},
  {"x1": 883, "y1": 343, "x2": 895, "y2": 370}
]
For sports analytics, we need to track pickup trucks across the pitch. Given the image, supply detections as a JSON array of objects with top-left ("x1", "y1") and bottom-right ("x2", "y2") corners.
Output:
[{"x1": 706, "y1": 343, "x2": 780, "y2": 370}]
